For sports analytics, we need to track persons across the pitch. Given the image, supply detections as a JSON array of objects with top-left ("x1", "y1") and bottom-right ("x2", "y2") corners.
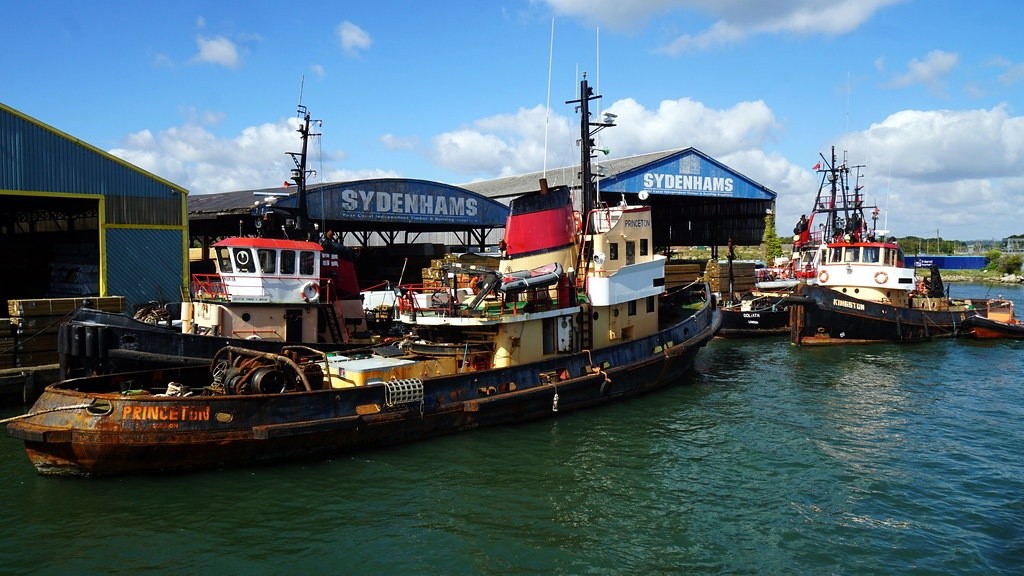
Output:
[{"x1": 819, "y1": 222, "x2": 905, "y2": 267}]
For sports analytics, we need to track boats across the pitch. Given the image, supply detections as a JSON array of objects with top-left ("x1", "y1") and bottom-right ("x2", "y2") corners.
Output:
[
  {"x1": 721, "y1": 144, "x2": 1024, "y2": 347},
  {"x1": 0, "y1": 72, "x2": 720, "y2": 475}
]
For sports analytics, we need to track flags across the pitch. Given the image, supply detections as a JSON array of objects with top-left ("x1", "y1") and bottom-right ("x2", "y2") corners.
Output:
[{"x1": 812, "y1": 160, "x2": 821, "y2": 170}]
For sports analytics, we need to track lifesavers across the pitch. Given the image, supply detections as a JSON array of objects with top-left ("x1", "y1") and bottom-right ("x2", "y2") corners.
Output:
[
  {"x1": 819, "y1": 270, "x2": 829, "y2": 283},
  {"x1": 874, "y1": 271, "x2": 888, "y2": 284},
  {"x1": 470, "y1": 275, "x2": 482, "y2": 294},
  {"x1": 300, "y1": 281, "x2": 321, "y2": 303}
]
